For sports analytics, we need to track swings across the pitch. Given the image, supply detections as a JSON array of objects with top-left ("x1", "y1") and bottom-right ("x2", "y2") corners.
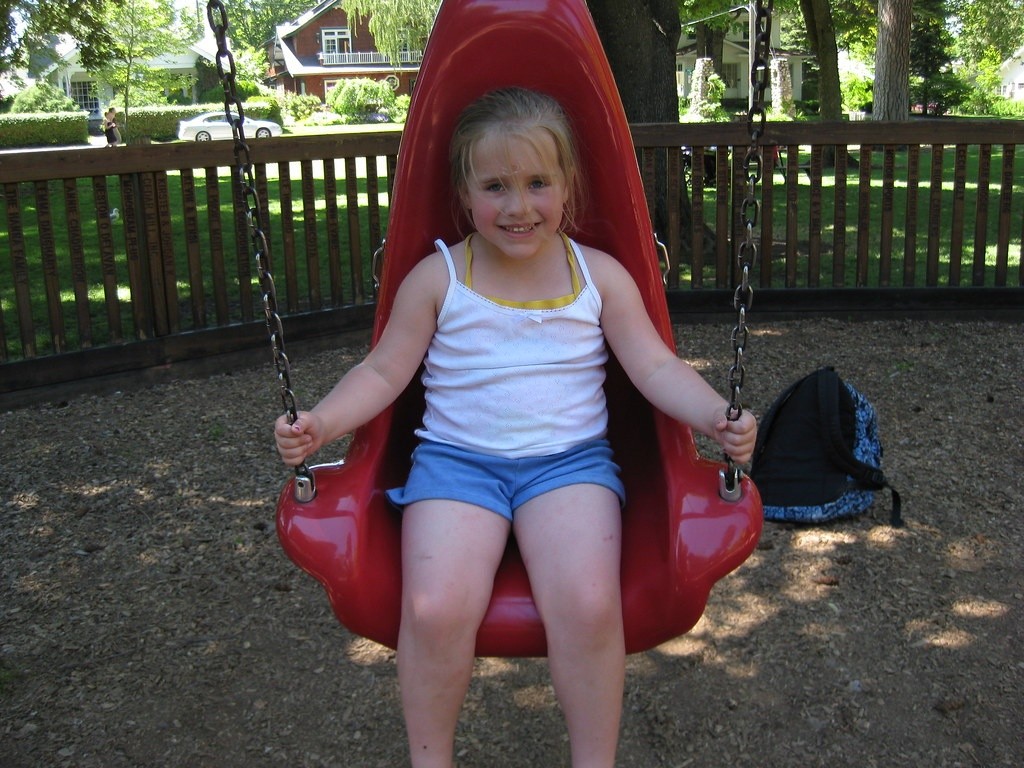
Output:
[{"x1": 203, "y1": 1, "x2": 769, "y2": 661}]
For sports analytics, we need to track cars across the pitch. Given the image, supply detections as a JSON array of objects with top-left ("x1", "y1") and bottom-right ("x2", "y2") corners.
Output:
[{"x1": 178, "y1": 111, "x2": 284, "y2": 143}]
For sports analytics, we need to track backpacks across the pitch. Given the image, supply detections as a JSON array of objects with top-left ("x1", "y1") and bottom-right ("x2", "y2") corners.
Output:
[{"x1": 745, "y1": 365, "x2": 904, "y2": 530}]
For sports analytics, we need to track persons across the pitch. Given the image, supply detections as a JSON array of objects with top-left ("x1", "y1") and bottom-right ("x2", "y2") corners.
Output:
[
  {"x1": 104, "y1": 107, "x2": 122, "y2": 147},
  {"x1": 273, "y1": 88, "x2": 758, "y2": 768}
]
